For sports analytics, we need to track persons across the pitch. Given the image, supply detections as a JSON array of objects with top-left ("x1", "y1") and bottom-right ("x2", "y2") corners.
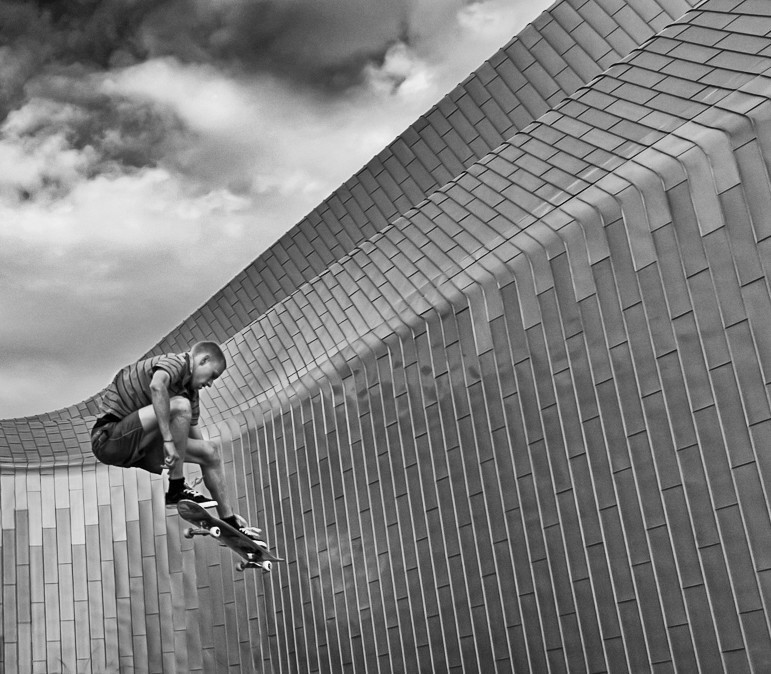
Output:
[{"x1": 90, "y1": 342, "x2": 269, "y2": 549}]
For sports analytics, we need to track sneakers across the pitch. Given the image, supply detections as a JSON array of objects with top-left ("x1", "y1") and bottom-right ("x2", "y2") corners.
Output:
[
  {"x1": 165, "y1": 486, "x2": 218, "y2": 509},
  {"x1": 236, "y1": 525, "x2": 267, "y2": 549}
]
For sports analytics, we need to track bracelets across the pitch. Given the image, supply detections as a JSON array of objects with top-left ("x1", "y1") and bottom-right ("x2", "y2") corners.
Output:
[{"x1": 163, "y1": 438, "x2": 175, "y2": 443}]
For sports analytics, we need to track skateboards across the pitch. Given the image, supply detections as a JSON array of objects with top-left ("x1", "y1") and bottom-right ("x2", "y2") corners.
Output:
[{"x1": 176, "y1": 499, "x2": 286, "y2": 574}]
